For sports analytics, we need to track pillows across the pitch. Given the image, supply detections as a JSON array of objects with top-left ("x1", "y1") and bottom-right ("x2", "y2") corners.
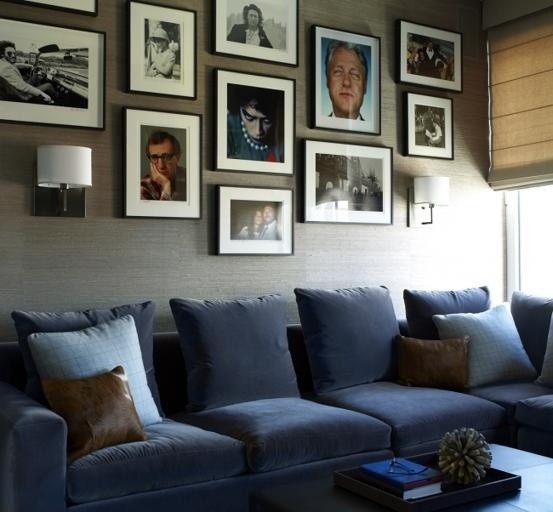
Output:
[
  {"x1": 535, "y1": 318, "x2": 552, "y2": 385},
  {"x1": 403, "y1": 288, "x2": 489, "y2": 341},
  {"x1": 296, "y1": 286, "x2": 405, "y2": 389},
  {"x1": 434, "y1": 308, "x2": 539, "y2": 390},
  {"x1": 11, "y1": 299, "x2": 160, "y2": 412},
  {"x1": 510, "y1": 292, "x2": 553, "y2": 367},
  {"x1": 30, "y1": 316, "x2": 164, "y2": 428},
  {"x1": 169, "y1": 296, "x2": 300, "y2": 412},
  {"x1": 394, "y1": 334, "x2": 470, "y2": 391},
  {"x1": 43, "y1": 368, "x2": 147, "y2": 463}
]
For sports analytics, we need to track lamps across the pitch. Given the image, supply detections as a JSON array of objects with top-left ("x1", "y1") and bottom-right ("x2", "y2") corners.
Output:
[
  {"x1": 33, "y1": 145, "x2": 93, "y2": 219},
  {"x1": 407, "y1": 175, "x2": 450, "y2": 227}
]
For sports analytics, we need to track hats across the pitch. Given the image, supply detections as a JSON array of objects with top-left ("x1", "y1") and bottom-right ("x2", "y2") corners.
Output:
[{"x1": 149, "y1": 28, "x2": 172, "y2": 43}]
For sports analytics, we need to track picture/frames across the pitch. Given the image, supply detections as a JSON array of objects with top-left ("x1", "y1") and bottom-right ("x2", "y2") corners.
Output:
[
  {"x1": 213, "y1": 0, "x2": 300, "y2": 68},
  {"x1": 309, "y1": 24, "x2": 382, "y2": 136},
  {"x1": 300, "y1": 138, "x2": 394, "y2": 226},
  {"x1": 0, "y1": 17, "x2": 107, "y2": 132},
  {"x1": 215, "y1": 69, "x2": 295, "y2": 176},
  {"x1": 15, "y1": 0, "x2": 98, "y2": 17},
  {"x1": 125, "y1": 109, "x2": 203, "y2": 220},
  {"x1": 216, "y1": 184, "x2": 295, "y2": 257},
  {"x1": 396, "y1": 20, "x2": 463, "y2": 92},
  {"x1": 126, "y1": 0, "x2": 198, "y2": 100},
  {"x1": 402, "y1": 89, "x2": 454, "y2": 161}
]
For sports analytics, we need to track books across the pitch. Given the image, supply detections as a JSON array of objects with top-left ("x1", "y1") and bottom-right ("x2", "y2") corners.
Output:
[{"x1": 359, "y1": 458, "x2": 445, "y2": 501}]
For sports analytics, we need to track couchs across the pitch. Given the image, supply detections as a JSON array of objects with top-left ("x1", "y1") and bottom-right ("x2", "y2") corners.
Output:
[{"x1": 1, "y1": 295, "x2": 552, "y2": 510}]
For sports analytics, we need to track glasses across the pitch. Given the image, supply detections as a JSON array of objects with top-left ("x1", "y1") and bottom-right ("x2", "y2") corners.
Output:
[
  {"x1": 384, "y1": 453, "x2": 429, "y2": 477},
  {"x1": 6, "y1": 51, "x2": 16, "y2": 57},
  {"x1": 147, "y1": 152, "x2": 176, "y2": 164}
]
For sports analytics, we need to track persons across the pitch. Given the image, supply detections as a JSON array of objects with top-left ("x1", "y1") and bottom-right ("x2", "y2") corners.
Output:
[
  {"x1": 415, "y1": 108, "x2": 444, "y2": 146},
  {"x1": 146, "y1": 28, "x2": 178, "y2": 78},
  {"x1": 0, "y1": 40, "x2": 61, "y2": 103},
  {"x1": 407, "y1": 33, "x2": 455, "y2": 81},
  {"x1": 320, "y1": 37, "x2": 372, "y2": 120},
  {"x1": 140, "y1": 131, "x2": 188, "y2": 202},
  {"x1": 232, "y1": 204, "x2": 281, "y2": 240},
  {"x1": 227, "y1": 83, "x2": 285, "y2": 163},
  {"x1": 227, "y1": 4, "x2": 275, "y2": 49}
]
